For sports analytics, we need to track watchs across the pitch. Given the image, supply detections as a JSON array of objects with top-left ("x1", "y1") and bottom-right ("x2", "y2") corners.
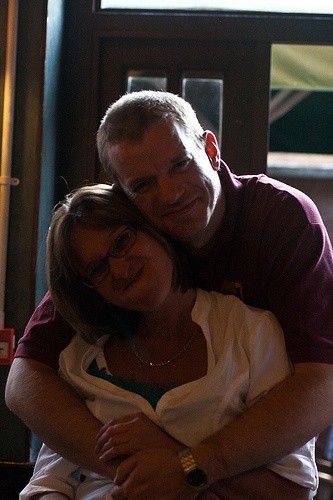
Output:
[{"x1": 176, "y1": 448, "x2": 211, "y2": 493}]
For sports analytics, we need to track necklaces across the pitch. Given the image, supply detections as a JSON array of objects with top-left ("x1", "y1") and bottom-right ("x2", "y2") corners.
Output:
[{"x1": 126, "y1": 318, "x2": 196, "y2": 369}]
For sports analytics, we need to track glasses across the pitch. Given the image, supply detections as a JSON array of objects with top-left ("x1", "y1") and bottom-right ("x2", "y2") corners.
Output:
[{"x1": 79, "y1": 224, "x2": 139, "y2": 289}]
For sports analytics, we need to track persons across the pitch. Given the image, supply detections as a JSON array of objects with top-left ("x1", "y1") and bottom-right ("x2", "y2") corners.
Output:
[
  {"x1": 1, "y1": 88, "x2": 333, "y2": 499},
  {"x1": 15, "y1": 181, "x2": 320, "y2": 499}
]
